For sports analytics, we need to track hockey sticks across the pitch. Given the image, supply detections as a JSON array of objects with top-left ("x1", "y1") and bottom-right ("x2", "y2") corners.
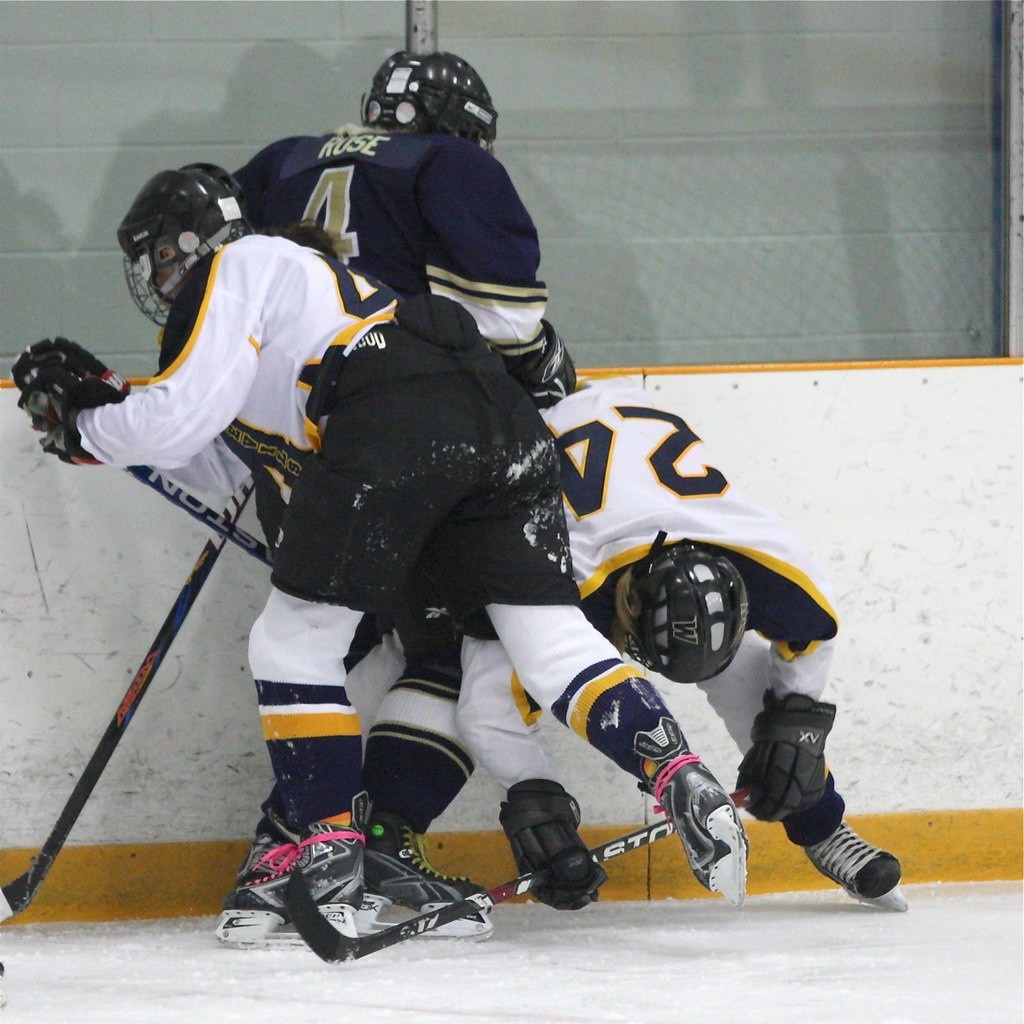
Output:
[
  {"x1": 0, "y1": 465, "x2": 258, "y2": 925},
  {"x1": 28, "y1": 391, "x2": 274, "y2": 568},
  {"x1": 281, "y1": 788, "x2": 754, "y2": 966}
]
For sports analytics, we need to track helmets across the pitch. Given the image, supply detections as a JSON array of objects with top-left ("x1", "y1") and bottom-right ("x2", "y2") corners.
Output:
[
  {"x1": 362, "y1": 49, "x2": 497, "y2": 153},
  {"x1": 116, "y1": 163, "x2": 248, "y2": 271},
  {"x1": 636, "y1": 530, "x2": 750, "y2": 686}
]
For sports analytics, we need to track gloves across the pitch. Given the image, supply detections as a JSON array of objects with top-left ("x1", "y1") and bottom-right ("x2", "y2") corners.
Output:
[
  {"x1": 500, "y1": 779, "x2": 606, "y2": 911},
  {"x1": 10, "y1": 337, "x2": 131, "y2": 467},
  {"x1": 734, "y1": 686, "x2": 837, "y2": 822},
  {"x1": 509, "y1": 315, "x2": 577, "y2": 408}
]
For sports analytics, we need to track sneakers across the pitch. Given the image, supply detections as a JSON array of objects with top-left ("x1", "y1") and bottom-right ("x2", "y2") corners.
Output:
[
  {"x1": 667, "y1": 762, "x2": 752, "y2": 908},
  {"x1": 353, "y1": 809, "x2": 495, "y2": 943},
  {"x1": 216, "y1": 822, "x2": 362, "y2": 952},
  {"x1": 806, "y1": 823, "x2": 906, "y2": 915}
]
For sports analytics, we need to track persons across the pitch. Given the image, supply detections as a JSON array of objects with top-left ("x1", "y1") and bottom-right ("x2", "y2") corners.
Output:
[
  {"x1": 216, "y1": 382, "x2": 909, "y2": 945},
  {"x1": 154, "y1": 50, "x2": 575, "y2": 931},
  {"x1": 12, "y1": 163, "x2": 748, "y2": 951}
]
